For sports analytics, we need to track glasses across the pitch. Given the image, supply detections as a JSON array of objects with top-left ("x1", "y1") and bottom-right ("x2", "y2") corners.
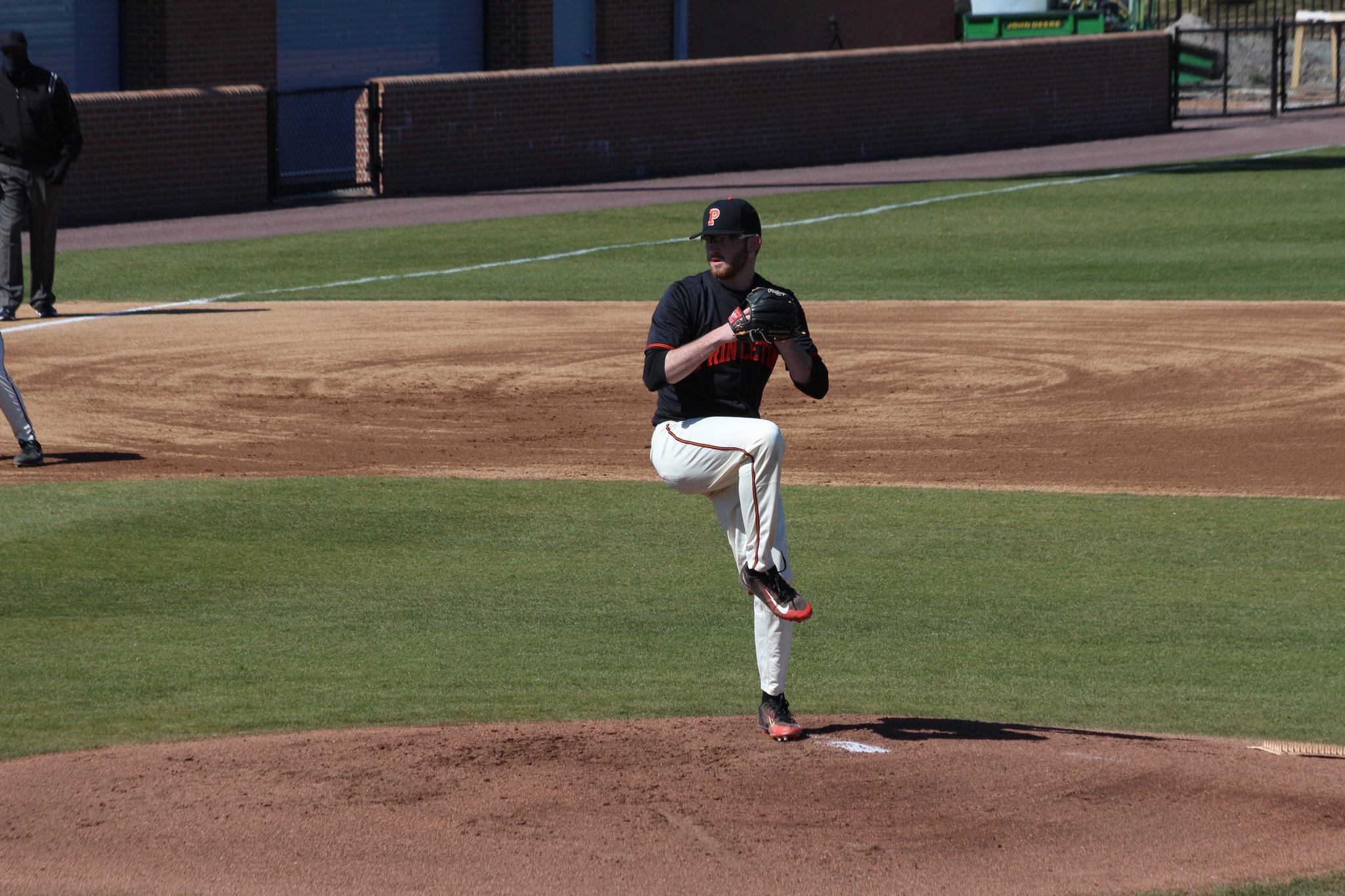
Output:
[{"x1": 701, "y1": 233, "x2": 758, "y2": 245}]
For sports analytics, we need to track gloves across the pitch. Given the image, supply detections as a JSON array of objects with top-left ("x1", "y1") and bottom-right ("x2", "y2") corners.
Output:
[{"x1": 43, "y1": 163, "x2": 68, "y2": 187}]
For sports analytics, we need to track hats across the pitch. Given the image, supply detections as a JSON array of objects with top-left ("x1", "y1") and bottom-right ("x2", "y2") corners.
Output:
[
  {"x1": 689, "y1": 197, "x2": 761, "y2": 240},
  {"x1": 0, "y1": 30, "x2": 27, "y2": 50}
]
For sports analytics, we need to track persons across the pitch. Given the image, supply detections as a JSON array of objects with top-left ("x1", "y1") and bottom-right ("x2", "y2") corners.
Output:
[
  {"x1": 638, "y1": 195, "x2": 829, "y2": 743},
  {"x1": 0, "y1": 27, "x2": 83, "y2": 320},
  {"x1": 0, "y1": 332, "x2": 45, "y2": 469}
]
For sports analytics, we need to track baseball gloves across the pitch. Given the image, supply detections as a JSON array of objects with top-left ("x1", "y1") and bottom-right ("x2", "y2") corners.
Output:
[{"x1": 726, "y1": 287, "x2": 806, "y2": 349}]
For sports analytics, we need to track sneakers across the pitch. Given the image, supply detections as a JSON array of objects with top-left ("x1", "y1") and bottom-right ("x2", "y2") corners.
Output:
[
  {"x1": 13, "y1": 440, "x2": 44, "y2": 467},
  {"x1": 0, "y1": 307, "x2": 17, "y2": 321},
  {"x1": 757, "y1": 689, "x2": 802, "y2": 741},
  {"x1": 35, "y1": 310, "x2": 59, "y2": 317},
  {"x1": 738, "y1": 561, "x2": 813, "y2": 624}
]
